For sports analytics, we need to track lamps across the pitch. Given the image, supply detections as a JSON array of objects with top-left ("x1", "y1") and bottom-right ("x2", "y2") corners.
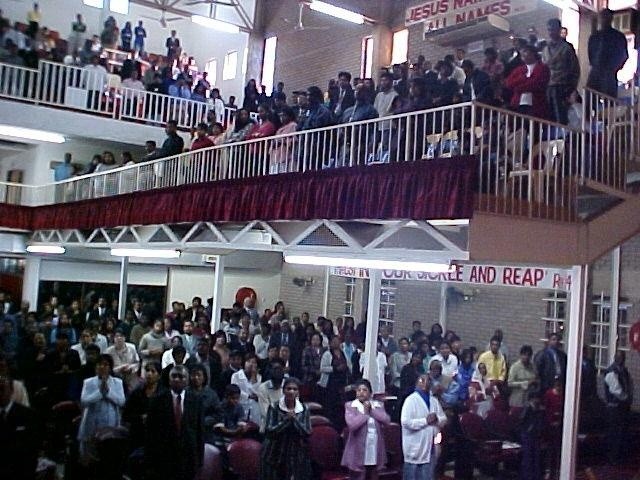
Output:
[
  {"x1": 306, "y1": 0, "x2": 368, "y2": 28},
  {"x1": 23, "y1": 241, "x2": 68, "y2": 259},
  {"x1": 284, "y1": 250, "x2": 460, "y2": 275},
  {"x1": 110, "y1": 246, "x2": 184, "y2": 263}
]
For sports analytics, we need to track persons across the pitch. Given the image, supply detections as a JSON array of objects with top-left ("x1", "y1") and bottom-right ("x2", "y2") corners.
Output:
[
  {"x1": 51, "y1": 1, "x2": 639, "y2": 202},
  {"x1": 1, "y1": 0, "x2": 238, "y2": 128},
  {"x1": 1, "y1": 287, "x2": 640, "y2": 478}
]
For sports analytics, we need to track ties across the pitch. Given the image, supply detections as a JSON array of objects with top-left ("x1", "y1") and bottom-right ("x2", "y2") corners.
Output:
[
  {"x1": 174, "y1": 396, "x2": 182, "y2": 428},
  {"x1": 282, "y1": 334, "x2": 286, "y2": 344}
]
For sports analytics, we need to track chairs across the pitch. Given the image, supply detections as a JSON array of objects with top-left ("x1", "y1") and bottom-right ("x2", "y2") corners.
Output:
[
  {"x1": 2, "y1": 13, "x2": 205, "y2": 100},
  {"x1": 430, "y1": 48, "x2": 639, "y2": 213},
  {"x1": 173, "y1": 386, "x2": 639, "y2": 478}
]
